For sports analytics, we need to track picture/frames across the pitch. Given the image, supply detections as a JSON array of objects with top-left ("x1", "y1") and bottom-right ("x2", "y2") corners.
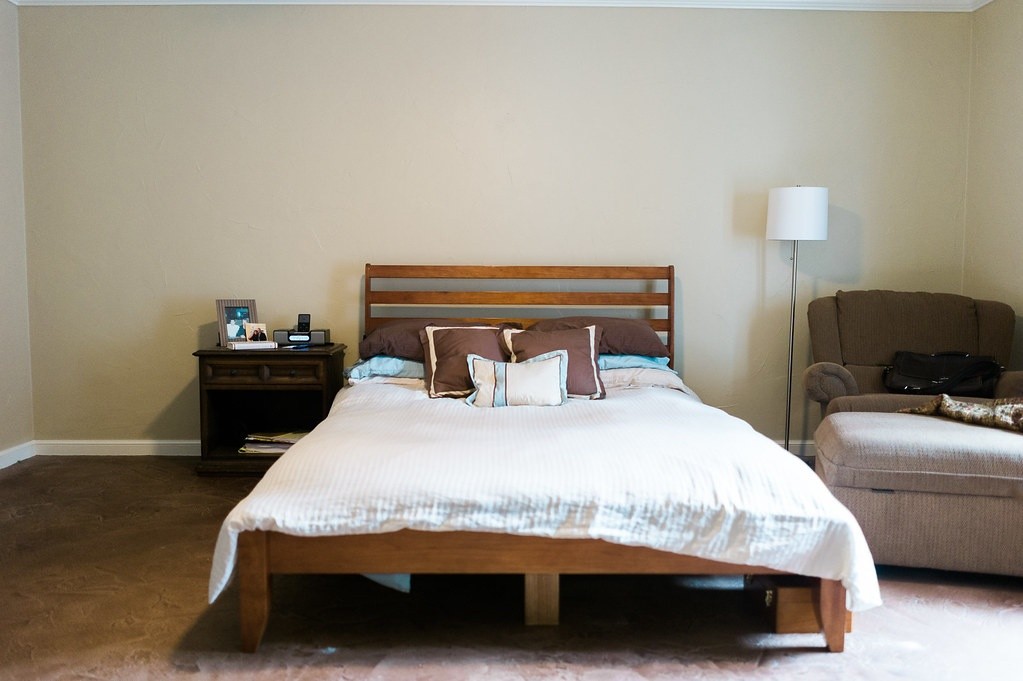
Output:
[
  {"x1": 216, "y1": 298, "x2": 258, "y2": 346},
  {"x1": 245, "y1": 322, "x2": 268, "y2": 342}
]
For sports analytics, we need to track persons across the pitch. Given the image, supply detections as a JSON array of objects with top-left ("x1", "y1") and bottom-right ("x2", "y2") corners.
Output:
[
  {"x1": 249, "y1": 327, "x2": 266, "y2": 341},
  {"x1": 228, "y1": 318, "x2": 250, "y2": 338}
]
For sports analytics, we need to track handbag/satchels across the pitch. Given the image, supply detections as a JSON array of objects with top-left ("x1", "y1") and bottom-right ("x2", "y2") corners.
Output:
[
  {"x1": 943, "y1": 354, "x2": 1007, "y2": 399},
  {"x1": 881, "y1": 350, "x2": 949, "y2": 395}
]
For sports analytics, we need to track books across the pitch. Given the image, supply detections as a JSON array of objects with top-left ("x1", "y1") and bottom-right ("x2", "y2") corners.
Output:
[
  {"x1": 238, "y1": 432, "x2": 309, "y2": 454},
  {"x1": 227, "y1": 341, "x2": 278, "y2": 350}
]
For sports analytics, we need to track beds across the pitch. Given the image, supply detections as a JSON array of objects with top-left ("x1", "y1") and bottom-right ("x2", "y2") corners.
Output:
[{"x1": 207, "y1": 258, "x2": 883, "y2": 653}]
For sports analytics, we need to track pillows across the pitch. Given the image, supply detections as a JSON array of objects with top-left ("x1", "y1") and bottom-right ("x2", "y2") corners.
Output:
[
  {"x1": 598, "y1": 353, "x2": 671, "y2": 371},
  {"x1": 418, "y1": 322, "x2": 510, "y2": 399},
  {"x1": 497, "y1": 323, "x2": 606, "y2": 400},
  {"x1": 599, "y1": 368, "x2": 686, "y2": 394},
  {"x1": 359, "y1": 320, "x2": 433, "y2": 362},
  {"x1": 526, "y1": 316, "x2": 671, "y2": 357},
  {"x1": 465, "y1": 349, "x2": 570, "y2": 405}
]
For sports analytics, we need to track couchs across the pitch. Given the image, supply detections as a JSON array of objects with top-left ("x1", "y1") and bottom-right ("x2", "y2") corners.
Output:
[{"x1": 803, "y1": 288, "x2": 1023, "y2": 424}]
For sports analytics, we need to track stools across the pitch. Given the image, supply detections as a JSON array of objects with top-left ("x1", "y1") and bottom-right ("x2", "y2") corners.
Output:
[{"x1": 812, "y1": 411, "x2": 1023, "y2": 578}]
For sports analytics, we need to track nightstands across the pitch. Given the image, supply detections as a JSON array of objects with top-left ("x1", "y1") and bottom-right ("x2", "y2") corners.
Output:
[{"x1": 191, "y1": 344, "x2": 348, "y2": 474}]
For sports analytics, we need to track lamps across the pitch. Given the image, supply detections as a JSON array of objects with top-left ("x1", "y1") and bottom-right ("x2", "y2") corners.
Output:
[{"x1": 766, "y1": 185, "x2": 829, "y2": 450}]
